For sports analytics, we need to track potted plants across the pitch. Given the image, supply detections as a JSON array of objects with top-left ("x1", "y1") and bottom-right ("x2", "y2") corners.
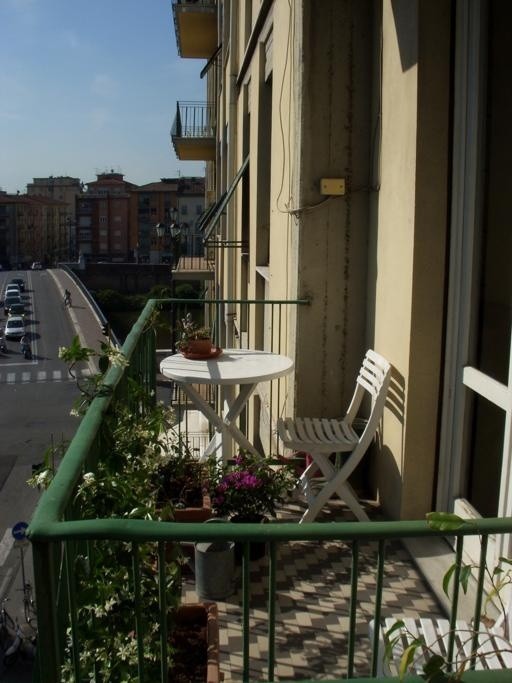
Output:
[{"x1": 175, "y1": 311, "x2": 213, "y2": 354}]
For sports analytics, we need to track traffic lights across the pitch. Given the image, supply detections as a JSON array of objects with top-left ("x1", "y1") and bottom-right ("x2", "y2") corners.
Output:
[
  {"x1": 32, "y1": 463, "x2": 42, "y2": 477},
  {"x1": 102, "y1": 323, "x2": 109, "y2": 336}
]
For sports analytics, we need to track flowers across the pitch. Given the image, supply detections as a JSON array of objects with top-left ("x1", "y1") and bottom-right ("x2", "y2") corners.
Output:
[{"x1": 207, "y1": 452, "x2": 281, "y2": 520}]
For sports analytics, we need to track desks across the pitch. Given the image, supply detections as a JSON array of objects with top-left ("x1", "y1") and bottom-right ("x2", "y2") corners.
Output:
[{"x1": 159, "y1": 347, "x2": 295, "y2": 477}]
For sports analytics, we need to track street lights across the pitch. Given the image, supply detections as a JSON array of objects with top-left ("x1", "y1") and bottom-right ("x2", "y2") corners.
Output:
[
  {"x1": 155, "y1": 204, "x2": 190, "y2": 353},
  {"x1": 66, "y1": 216, "x2": 77, "y2": 262}
]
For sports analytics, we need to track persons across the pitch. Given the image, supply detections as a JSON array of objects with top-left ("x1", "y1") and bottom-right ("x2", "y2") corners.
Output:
[
  {"x1": 20, "y1": 334, "x2": 32, "y2": 353},
  {"x1": 64, "y1": 288, "x2": 71, "y2": 305}
]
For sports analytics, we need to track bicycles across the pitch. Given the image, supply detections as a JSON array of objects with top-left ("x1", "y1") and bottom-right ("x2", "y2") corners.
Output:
[{"x1": 1, "y1": 581, "x2": 39, "y2": 635}]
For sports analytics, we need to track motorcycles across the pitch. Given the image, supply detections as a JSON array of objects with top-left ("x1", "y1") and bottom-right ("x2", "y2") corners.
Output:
[
  {"x1": 21, "y1": 340, "x2": 32, "y2": 359},
  {"x1": 0, "y1": 335, "x2": 8, "y2": 352}
]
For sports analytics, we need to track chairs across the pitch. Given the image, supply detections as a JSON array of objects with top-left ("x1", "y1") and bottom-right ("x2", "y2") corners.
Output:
[{"x1": 277, "y1": 348, "x2": 394, "y2": 523}]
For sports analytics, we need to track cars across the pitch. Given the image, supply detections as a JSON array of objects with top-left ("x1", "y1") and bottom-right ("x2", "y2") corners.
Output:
[
  {"x1": 0, "y1": 276, "x2": 26, "y2": 339},
  {"x1": 32, "y1": 261, "x2": 42, "y2": 269}
]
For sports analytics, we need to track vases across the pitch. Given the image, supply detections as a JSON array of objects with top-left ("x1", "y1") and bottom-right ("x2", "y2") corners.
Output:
[{"x1": 230, "y1": 513, "x2": 272, "y2": 560}]
